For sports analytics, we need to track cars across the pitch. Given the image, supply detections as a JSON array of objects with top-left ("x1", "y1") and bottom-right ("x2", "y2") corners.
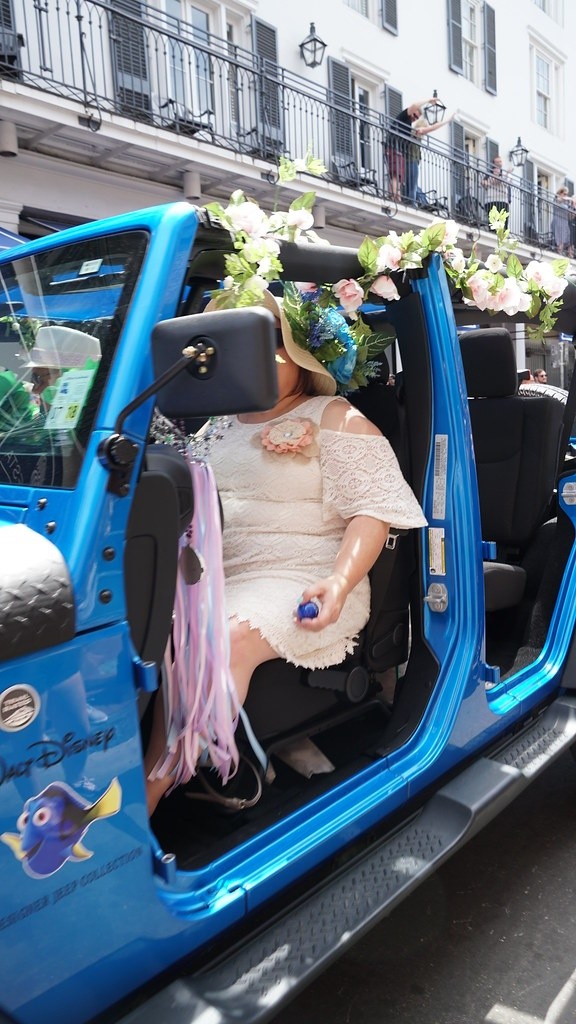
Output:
[{"x1": 0, "y1": 201, "x2": 575, "y2": 1024}]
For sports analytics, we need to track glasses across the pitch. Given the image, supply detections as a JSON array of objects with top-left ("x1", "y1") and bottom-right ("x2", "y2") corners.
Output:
[
  {"x1": 32, "y1": 371, "x2": 56, "y2": 390},
  {"x1": 413, "y1": 113, "x2": 418, "y2": 119},
  {"x1": 276, "y1": 328, "x2": 284, "y2": 348},
  {"x1": 538, "y1": 375, "x2": 547, "y2": 377}
]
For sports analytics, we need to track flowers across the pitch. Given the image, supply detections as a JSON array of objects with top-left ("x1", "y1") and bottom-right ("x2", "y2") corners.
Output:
[{"x1": 200, "y1": 151, "x2": 573, "y2": 392}]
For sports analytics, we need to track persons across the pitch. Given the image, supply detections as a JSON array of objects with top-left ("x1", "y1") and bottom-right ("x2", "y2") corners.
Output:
[
  {"x1": 137, "y1": 284, "x2": 428, "y2": 819},
  {"x1": 385, "y1": 97, "x2": 440, "y2": 203},
  {"x1": 551, "y1": 186, "x2": 576, "y2": 260},
  {"x1": 518, "y1": 369, "x2": 547, "y2": 384},
  {"x1": 20, "y1": 326, "x2": 102, "y2": 416},
  {"x1": 402, "y1": 113, "x2": 456, "y2": 208},
  {"x1": 481, "y1": 157, "x2": 511, "y2": 236}
]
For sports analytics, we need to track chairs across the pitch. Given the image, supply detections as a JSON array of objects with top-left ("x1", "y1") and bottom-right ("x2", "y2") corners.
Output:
[
  {"x1": 331, "y1": 155, "x2": 379, "y2": 195},
  {"x1": 235, "y1": 326, "x2": 566, "y2": 756},
  {"x1": 523, "y1": 222, "x2": 558, "y2": 252},
  {"x1": 233, "y1": 124, "x2": 291, "y2": 165},
  {"x1": 457, "y1": 195, "x2": 489, "y2": 231},
  {"x1": 400, "y1": 185, "x2": 450, "y2": 219},
  {"x1": 156, "y1": 96, "x2": 219, "y2": 144},
  {"x1": 0, "y1": 26, "x2": 25, "y2": 82}
]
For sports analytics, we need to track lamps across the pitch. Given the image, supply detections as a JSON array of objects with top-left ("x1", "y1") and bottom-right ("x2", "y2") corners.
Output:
[
  {"x1": 511, "y1": 137, "x2": 529, "y2": 166},
  {"x1": 423, "y1": 89, "x2": 446, "y2": 125},
  {"x1": 300, "y1": 21, "x2": 327, "y2": 67}
]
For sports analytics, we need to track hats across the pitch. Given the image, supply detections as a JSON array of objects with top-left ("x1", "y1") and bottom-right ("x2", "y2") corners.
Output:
[
  {"x1": 203, "y1": 286, "x2": 337, "y2": 399},
  {"x1": 19, "y1": 326, "x2": 102, "y2": 369}
]
